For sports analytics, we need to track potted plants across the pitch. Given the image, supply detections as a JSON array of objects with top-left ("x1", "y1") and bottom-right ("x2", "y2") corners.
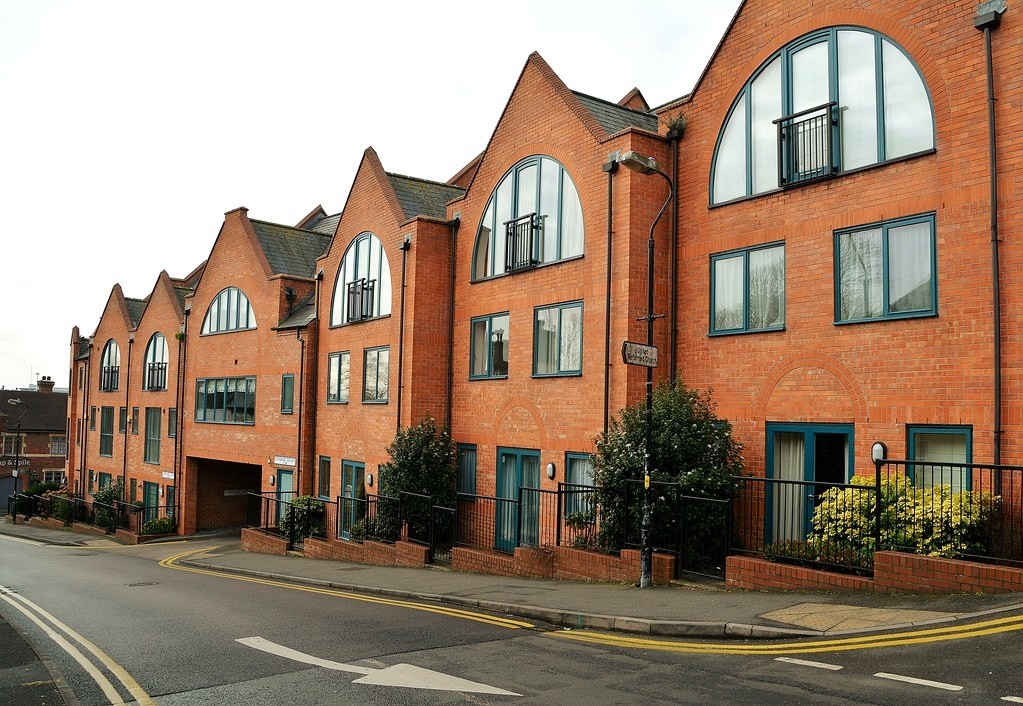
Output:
[{"x1": 661, "y1": 109, "x2": 687, "y2": 142}]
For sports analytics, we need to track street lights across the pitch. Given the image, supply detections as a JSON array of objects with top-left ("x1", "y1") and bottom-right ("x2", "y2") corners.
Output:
[
  {"x1": 620, "y1": 149, "x2": 674, "y2": 586},
  {"x1": 8, "y1": 398, "x2": 31, "y2": 525}
]
